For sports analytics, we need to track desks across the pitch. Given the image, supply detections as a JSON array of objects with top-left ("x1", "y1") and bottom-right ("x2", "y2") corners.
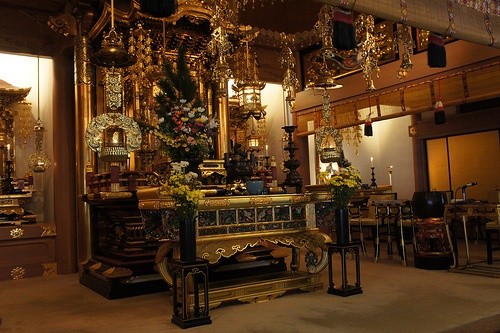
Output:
[
  {"x1": 79, "y1": 191, "x2": 287, "y2": 300},
  {"x1": 368, "y1": 200, "x2": 416, "y2": 266},
  {"x1": 305, "y1": 183, "x2": 371, "y2": 256},
  {"x1": 444, "y1": 203, "x2": 500, "y2": 277}
]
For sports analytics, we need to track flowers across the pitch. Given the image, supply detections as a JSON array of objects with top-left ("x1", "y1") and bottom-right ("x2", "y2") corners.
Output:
[
  {"x1": 133, "y1": 40, "x2": 220, "y2": 221},
  {"x1": 315, "y1": 161, "x2": 363, "y2": 208}
]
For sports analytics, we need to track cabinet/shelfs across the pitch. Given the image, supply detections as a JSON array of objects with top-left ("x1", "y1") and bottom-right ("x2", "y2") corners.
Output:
[{"x1": 0, "y1": 223, "x2": 57, "y2": 281}]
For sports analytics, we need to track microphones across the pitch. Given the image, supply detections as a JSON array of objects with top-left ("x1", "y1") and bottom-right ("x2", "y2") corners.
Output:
[{"x1": 459, "y1": 181, "x2": 478, "y2": 188}]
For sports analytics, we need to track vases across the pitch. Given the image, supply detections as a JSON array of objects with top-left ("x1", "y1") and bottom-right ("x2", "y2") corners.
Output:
[
  {"x1": 178, "y1": 221, "x2": 197, "y2": 264},
  {"x1": 335, "y1": 208, "x2": 350, "y2": 245}
]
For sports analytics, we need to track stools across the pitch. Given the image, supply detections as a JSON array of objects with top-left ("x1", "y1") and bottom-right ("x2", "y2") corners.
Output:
[
  {"x1": 171, "y1": 256, "x2": 212, "y2": 329},
  {"x1": 326, "y1": 244, "x2": 363, "y2": 297}
]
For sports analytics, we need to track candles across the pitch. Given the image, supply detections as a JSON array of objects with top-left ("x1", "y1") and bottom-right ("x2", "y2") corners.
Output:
[
  {"x1": 388, "y1": 171, "x2": 392, "y2": 186},
  {"x1": 287, "y1": 100, "x2": 293, "y2": 126},
  {"x1": 265, "y1": 144, "x2": 269, "y2": 156},
  {"x1": 6, "y1": 145, "x2": 10, "y2": 160},
  {"x1": 370, "y1": 156, "x2": 374, "y2": 167}
]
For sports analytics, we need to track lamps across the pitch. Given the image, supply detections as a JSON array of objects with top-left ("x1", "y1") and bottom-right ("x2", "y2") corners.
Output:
[{"x1": 27, "y1": 0, "x2": 446, "y2": 172}]
